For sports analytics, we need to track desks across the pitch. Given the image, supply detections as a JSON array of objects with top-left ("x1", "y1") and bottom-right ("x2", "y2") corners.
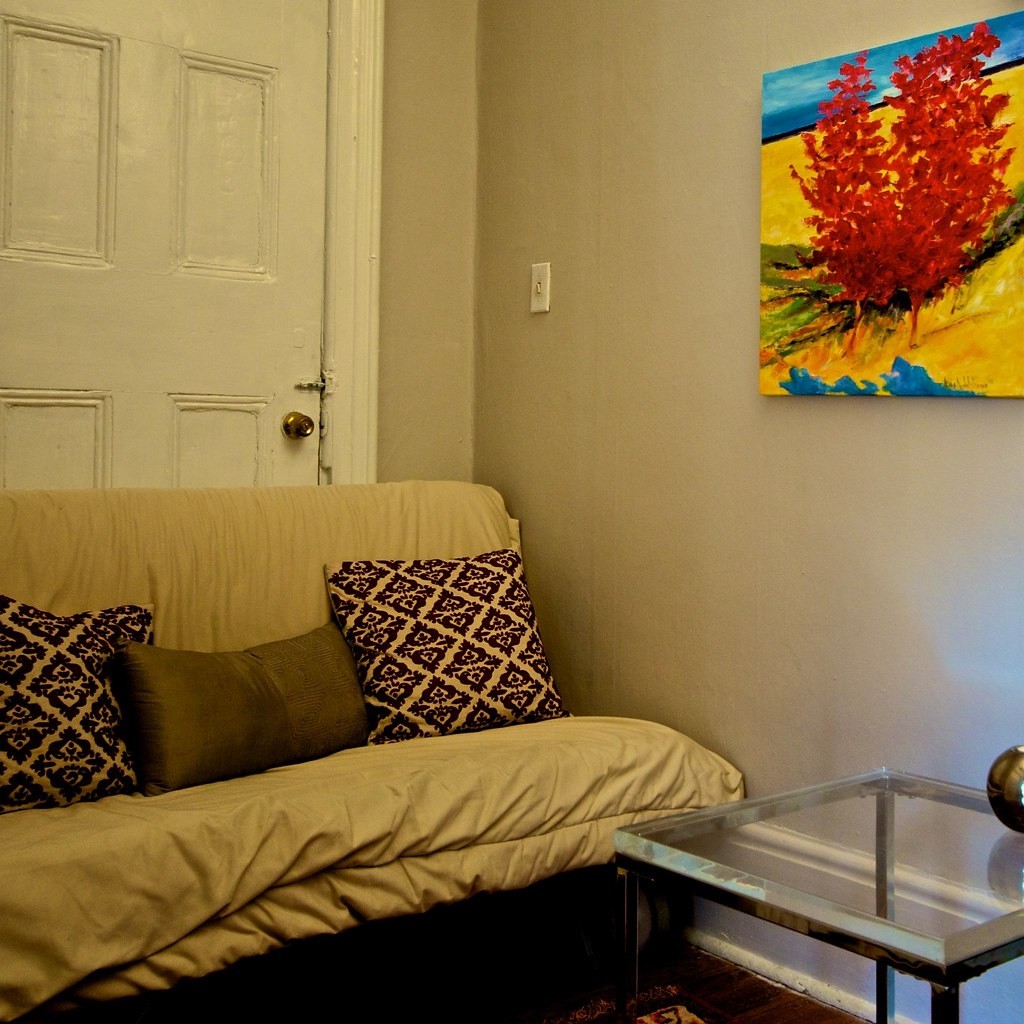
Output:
[{"x1": 613, "y1": 766, "x2": 1024, "y2": 1024}]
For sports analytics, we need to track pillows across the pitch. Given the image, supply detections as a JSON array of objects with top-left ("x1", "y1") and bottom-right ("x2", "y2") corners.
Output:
[
  {"x1": 117, "y1": 621, "x2": 369, "y2": 798},
  {"x1": 324, "y1": 547, "x2": 575, "y2": 746},
  {"x1": 0, "y1": 591, "x2": 156, "y2": 815}
]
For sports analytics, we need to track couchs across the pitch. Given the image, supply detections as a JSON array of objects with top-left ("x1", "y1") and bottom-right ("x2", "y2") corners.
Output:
[{"x1": 0, "y1": 481, "x2": 747, "y2": 1024}]
